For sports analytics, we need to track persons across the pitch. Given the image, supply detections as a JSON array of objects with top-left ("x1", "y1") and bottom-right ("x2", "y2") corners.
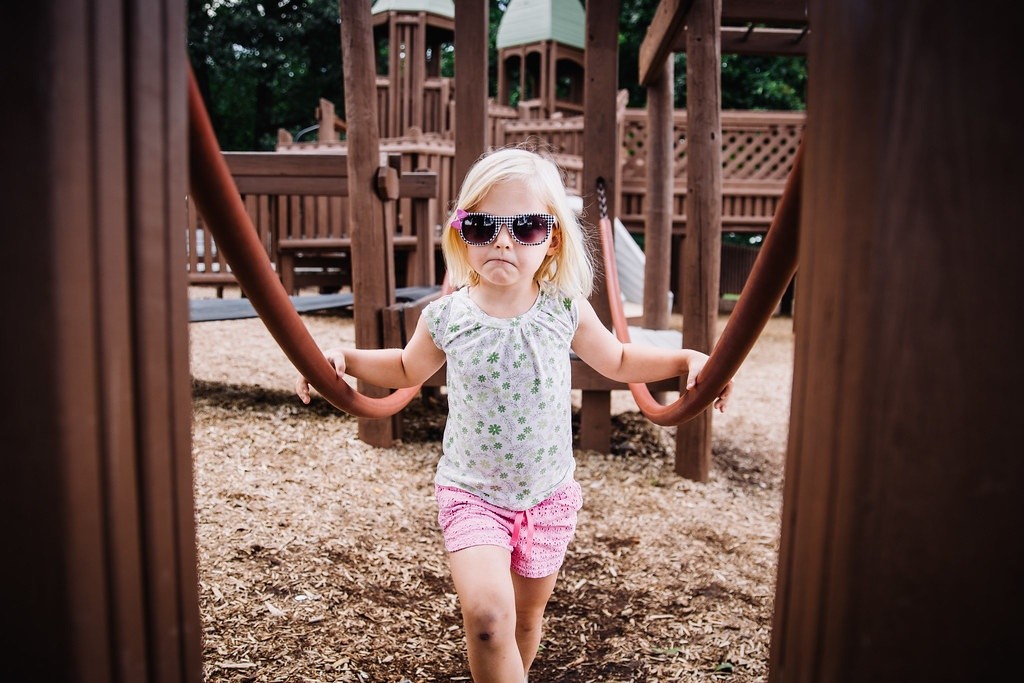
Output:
[{"x1": 295, "y1": 147, "x2": 733, "y2": 683}]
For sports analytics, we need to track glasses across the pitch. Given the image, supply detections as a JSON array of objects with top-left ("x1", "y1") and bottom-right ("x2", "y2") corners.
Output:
[{"x1": 458, "y1": 212, "x2": 559, "y2": 247}]
